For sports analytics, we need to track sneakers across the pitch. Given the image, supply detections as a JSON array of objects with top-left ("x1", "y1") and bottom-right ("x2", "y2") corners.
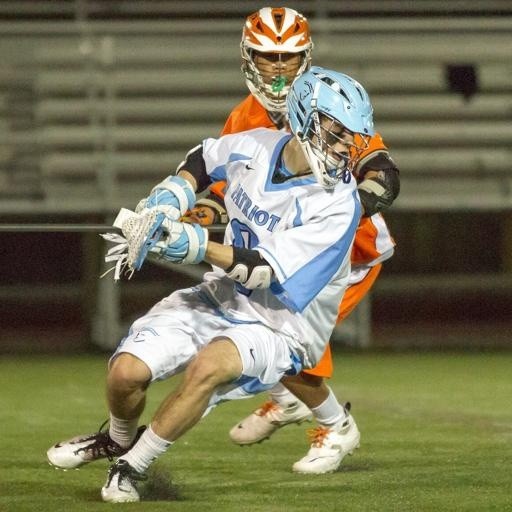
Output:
[
  {"x1": 102, "y1": 459, "x2": 149, "y2": 502},
  {"x1": 292, "y1": 402, "x2": 361, "y2": 474},
  {"x1": 46, "y1": 417, "x2": 147, "y2": 472},
  {"x1": 230, "y1": 398, "x2": 315, "y2": 446}
]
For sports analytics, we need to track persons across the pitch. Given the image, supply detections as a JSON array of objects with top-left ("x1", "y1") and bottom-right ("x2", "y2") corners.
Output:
[
  {"x1": 42, "y1": 64, "x2": 376, "y2": 506},
  {"x1": 170, "y1": 6, "x2": 399, "y2": 476}
]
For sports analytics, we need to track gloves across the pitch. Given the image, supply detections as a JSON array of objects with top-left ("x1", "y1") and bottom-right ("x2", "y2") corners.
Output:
[{"x1": 135, "y1": 174, "x2": 228, "y2": 265}]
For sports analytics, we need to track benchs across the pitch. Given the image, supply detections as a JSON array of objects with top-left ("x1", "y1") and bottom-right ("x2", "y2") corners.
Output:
[{"x1": 0, "y1": 2, "x2": 510, "y2": 216}]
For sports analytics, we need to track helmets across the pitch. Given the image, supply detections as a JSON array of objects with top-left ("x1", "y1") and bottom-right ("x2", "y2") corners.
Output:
[
  {"x1": 239, "y1": 7, "x2": 315, "y2": 112},
  {"x1": 285, "y1": 67, "x2": 374, "y2": 190}
]
